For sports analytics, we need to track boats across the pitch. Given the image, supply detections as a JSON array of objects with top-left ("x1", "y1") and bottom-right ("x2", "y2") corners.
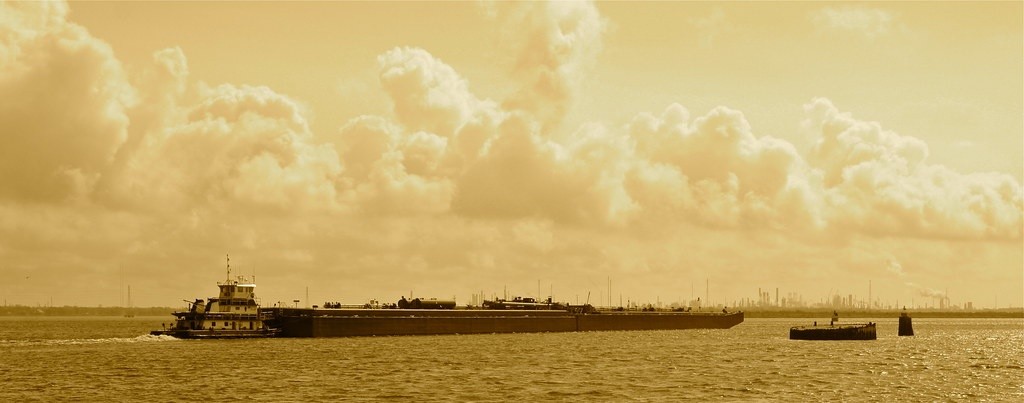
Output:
[{"x1": 151, "y1": 250, "x2": 280, "y2": 338}]
